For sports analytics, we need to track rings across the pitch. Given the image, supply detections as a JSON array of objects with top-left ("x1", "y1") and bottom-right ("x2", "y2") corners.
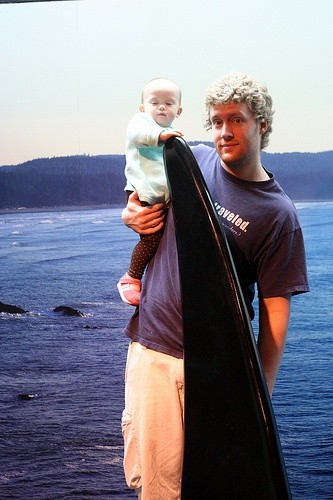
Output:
[{"x1": 152, "y1": 221, "x2": 155, "y2": 228}]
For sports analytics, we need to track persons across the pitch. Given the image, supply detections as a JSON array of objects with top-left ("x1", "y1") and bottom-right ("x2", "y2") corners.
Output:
[
  {"x1": 116, "y1": 74, "x2": 311, "y2": 500},
  {"x1": 117, "y1": 76, "x2": 186, "y2": 305}
]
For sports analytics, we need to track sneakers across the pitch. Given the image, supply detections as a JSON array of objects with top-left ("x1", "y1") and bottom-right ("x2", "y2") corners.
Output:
[{"x1": 116, "y1": 272, "x2": 142, "y2": 307}]
